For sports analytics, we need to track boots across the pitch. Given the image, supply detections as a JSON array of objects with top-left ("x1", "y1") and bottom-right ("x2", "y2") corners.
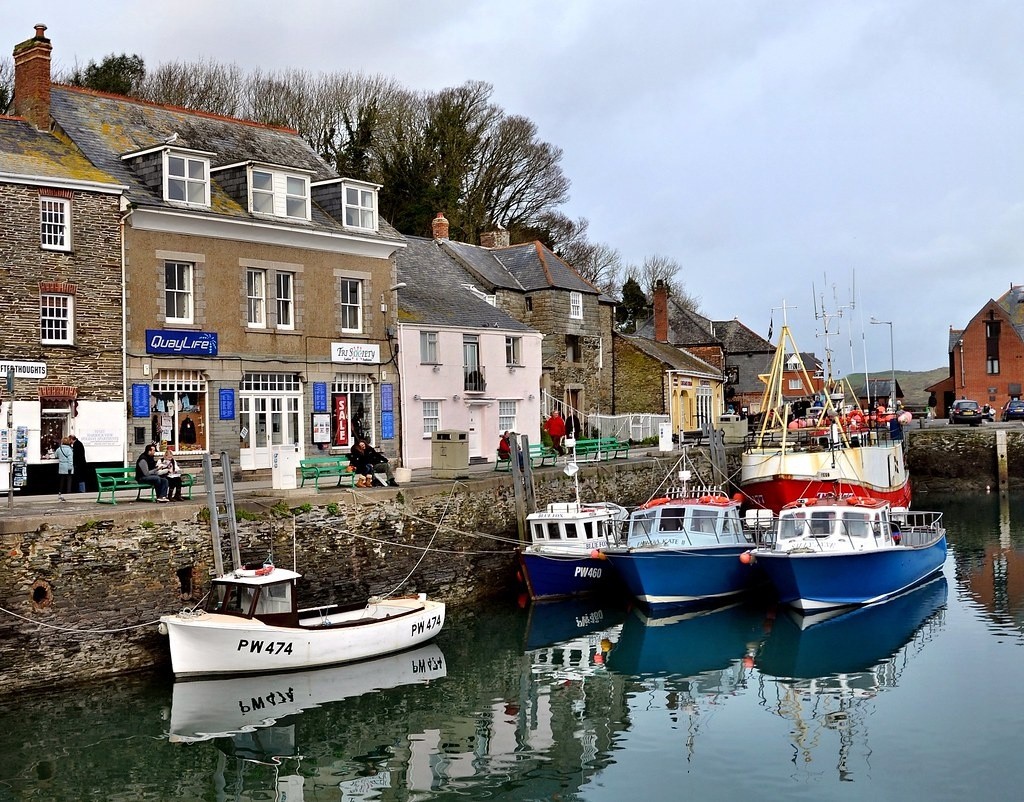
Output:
[
  {"x1": 356, "y1": 474, "x2": 366, "y2": 488},
  {"x1": 366, "y1": 474, "x2": 373, "y2": 488},
  {"x1": 175, "y1": 489, "x2": 184, "y2": 501},
  {"x1": 168, "y1": 489, "x2": 176, "y2": 502}
]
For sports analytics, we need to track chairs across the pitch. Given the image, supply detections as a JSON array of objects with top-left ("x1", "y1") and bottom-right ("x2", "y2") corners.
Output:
[{"x1": 960, "y1": 403, "x2": 964, "y2": 407}]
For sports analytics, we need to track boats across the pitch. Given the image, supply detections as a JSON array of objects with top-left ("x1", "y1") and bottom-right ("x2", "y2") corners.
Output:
[
  {"x1": 605, "y1": 599, "x2": 755, "y2": 705},
  {"x1": 748, "y1": 573, "x2": 949, "y2": 782},
  {"x1": 168, "y1": 639, "x2": 447, "y2": 764},
  {"x1": 788, "y1": 410, "x2": 911, "y2": 430},
  {"x1": 160, "y1": 561, "x2": 445, "y2": 679},
  {"x1": 747, "y1": 479, "x2": 948, "y2": 611},
  {"x1": 740, "y1": 298, "x2": 913, "y2": 516},
  {"x1": 518, "y1": 596, "x2": 627, "y2": 684},
  {"x1": 520, "y1": 500, "x2": 629, "y2": 601},
  {"x1": 602, "y1": 483, "x2": 779, "y2": 611}
]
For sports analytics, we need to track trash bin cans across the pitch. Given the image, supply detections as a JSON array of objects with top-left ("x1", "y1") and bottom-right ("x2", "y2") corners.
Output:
[
  {"x1": 431, "y1": 429, "x2": 470, "y2": 478},
  {"x1": 717, "y1": 414, "x2": 741, "y2": 443},
  {"x1": 806, "y1": 407, "x2": 824, "y2": 417}
]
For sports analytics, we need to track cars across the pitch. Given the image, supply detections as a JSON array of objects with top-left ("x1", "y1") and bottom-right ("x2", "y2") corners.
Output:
[
  {"x1": 949, "y1": 400, "x2": 982, "y2": 425},
  {"x1": 1001, "y1": 397, "x2": 1024, "y2": 421}
]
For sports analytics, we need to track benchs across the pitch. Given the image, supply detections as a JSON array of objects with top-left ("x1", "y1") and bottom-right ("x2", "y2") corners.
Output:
[
  {"x1": 299, "y1": 452, "x2": 387, "y2": 491},
  {"x1": 493, "y1": 444, "x2": 558, "y2": 471},
  {"x1": 674, "y1": 427, "x2": 725, "y2": 448},
  {"x1": 575, "y1": 437, "x2": 630, "y2": 462},
  {"x1": 95, "y1": 467, "x2": 194, "y2": 505}
]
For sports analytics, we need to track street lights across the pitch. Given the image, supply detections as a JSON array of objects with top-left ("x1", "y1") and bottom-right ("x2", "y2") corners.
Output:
[{"x1": 882, "y1": 322, "x2": 897, "y2": 407}]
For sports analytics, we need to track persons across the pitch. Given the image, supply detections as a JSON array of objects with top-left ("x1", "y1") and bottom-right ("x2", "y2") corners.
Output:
[
  {"x1": 928, "y1": 391, "x2": 937, "y2": 422},
  {"x1": 499, "y1": 430, "x2": 527, "y2": 472},
  {"x1": 54, "y1": 434, "x2": 88, "y2": 494},
  {"x1": 155, "y1": 450, "x2": 184, "y2": 502},
  {"x1": 787, "y1": 394, "x2": 823, "y2": 419},
  {"x1": 135, "y1": 445, "x2": 170, "y2": 502},
  {"x1": 349, "y1": 440, "x2": 399, "y2": 487},
  {"x1": 565, "y1": 410, "x2": 581, "y2": 458},
  {"x1": 543, "y1": 410, "x2": 565, "y2": 455}
]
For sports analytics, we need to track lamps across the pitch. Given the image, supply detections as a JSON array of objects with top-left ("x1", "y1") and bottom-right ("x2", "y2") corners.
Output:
[
  {"x1": 433, "y1": 366, "x2": 441, "y2": 373},
  {"x1": 415, "y1": 394, "x2": 421, "y2": 401},
  {"x1": 297, "y1": 375, "x2": 308, "y2": 384},
  {"x1": 493, "y1": 322, "x2": 500, "y2": 328},
  {"x1": 482, "y1": 323, "x2": 489, "y2": 327},
  {"x1": 382, "y1": 282, "x2": 407, "y2": 294},
  {"x1": 453, "y1": 394, "x2": 461, "y2": 402},
  {"x1": 527, "y1": 394, "x2": 535, "y2": 400}
]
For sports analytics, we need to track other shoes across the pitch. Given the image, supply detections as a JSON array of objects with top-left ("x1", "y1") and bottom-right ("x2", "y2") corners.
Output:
[
  {"x1": 389, "y1": 479, "x2": 399, "y2": 487},
  {"x1": 156, "y1": 496, "x2": 169, "y2": 502},
  {"x1": 375, "y1": 478, "x2": 388, "y2": 486}
]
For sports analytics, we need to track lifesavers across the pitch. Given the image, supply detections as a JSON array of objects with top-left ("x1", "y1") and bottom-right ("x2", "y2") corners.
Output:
[
  {"x1": 700, "y1": 496, "x2": 729, "y2": 503},
  {"x1": 846, "y1": 497, "x2": 877, "y2": 505},
  {"x1": 782, "y1": 498, "x2": 817, "y2": 509},
  {"x1": 847, "y1": 411, "x2": 865, "y2": 430},
  {"x1": 640, "y1": 498, "x2": 671, "y2": 509},
  {"x1": 235, "y1": 564, "x2": 273, "y2": 576}
]
